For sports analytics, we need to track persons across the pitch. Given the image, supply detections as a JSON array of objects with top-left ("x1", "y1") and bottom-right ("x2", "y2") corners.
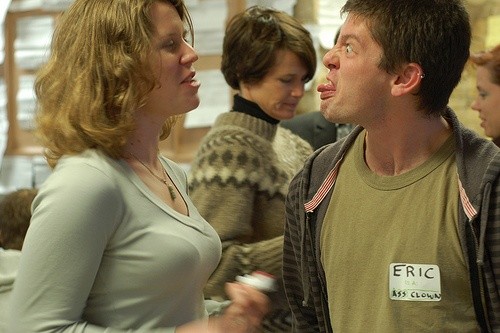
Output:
[
  {"x1": 187, "y1": 6, "x2": 317, "y2": 333},
  {"x1": 468, "y1": 46, "x2": 500, "y2": 148},
  {"x1": 8, "y1": 0, "x2": 269, "y2": 333},
  {"x1": 282, "y1": 0, "x2": 500, "y2": 333}
]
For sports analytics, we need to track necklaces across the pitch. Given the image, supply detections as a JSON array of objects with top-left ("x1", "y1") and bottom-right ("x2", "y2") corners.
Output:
[{"x1": 127, "y1": 150, "x2": 175, "y2": 200}]
